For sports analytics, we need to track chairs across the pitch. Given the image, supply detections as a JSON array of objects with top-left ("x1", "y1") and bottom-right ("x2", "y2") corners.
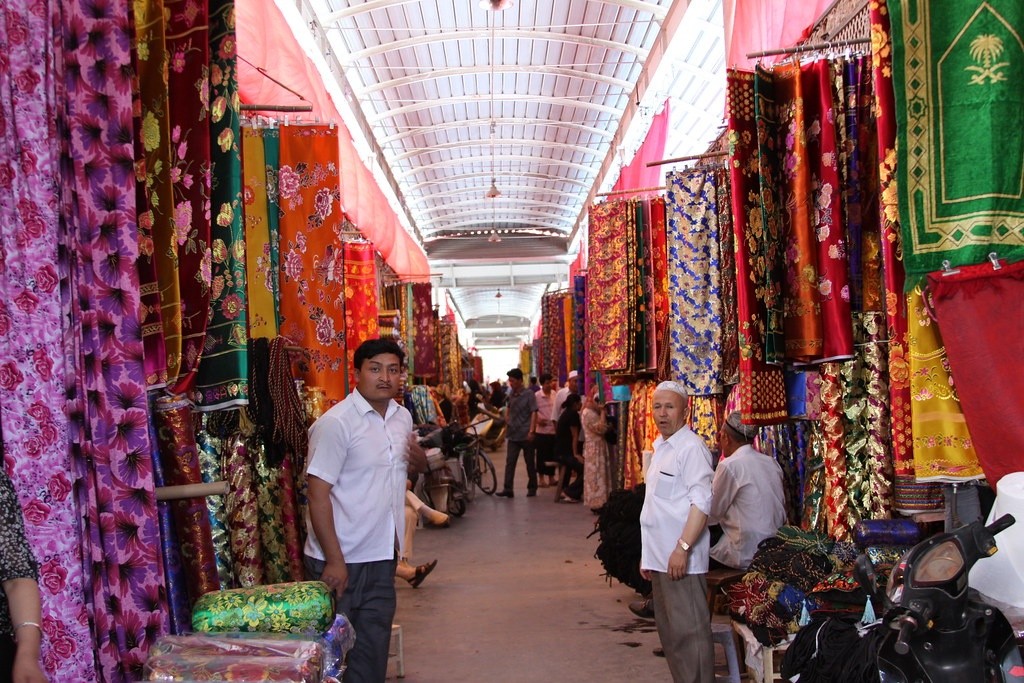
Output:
[{"x1": 388, "y1": 625, "x2": 404, "y2": 679}]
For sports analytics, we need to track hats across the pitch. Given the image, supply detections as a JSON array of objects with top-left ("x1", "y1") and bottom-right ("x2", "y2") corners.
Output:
[
  {"x1": 569, "y1": 371, "x2": 578, "y2": 377},
  {"x1": 726, "y1": 413, "x2": 759, "y2": 439},
  {"x1": 655, "y1": 381, "x2": 688, "y2": 401}
]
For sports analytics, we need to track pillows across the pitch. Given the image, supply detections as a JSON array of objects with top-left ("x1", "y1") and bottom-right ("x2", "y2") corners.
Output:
[
  {"x1": 853, "y1": 519, "x2": 920, "y2": 580},
  {"x1": 149, "y1": 581, "x2": 348, "y2": 683}
]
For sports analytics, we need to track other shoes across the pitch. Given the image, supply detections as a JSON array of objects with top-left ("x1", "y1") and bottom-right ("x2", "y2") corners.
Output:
[
  {"x1": 423, "y1": 508, "x2": 449, "y2": 524},
  {"x1": 560, "y1": 493, "x2": 578, "y2": 503},
  {"x1": 527, "y1": 487, "x2": 537, "y2": 499},
  {"x1": 628, "y1": 600, "x2": 655, "y2": 617},
  {"x1": 495, "y1": 490, "x2": 515, "y2": 499},
  {"x1": 539, "y1": 481, "x2": 550, "y2": 487},
  {"x1": 653, "y1": 646, "x2": 667, "y2": 656},
  {"x1": 549, "y1": 479, "x2": 558, "y2": 484}
]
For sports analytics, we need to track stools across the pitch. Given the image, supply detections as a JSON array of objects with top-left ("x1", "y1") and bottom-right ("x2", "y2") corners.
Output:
[{"x1": 711, "y1": 623, "x2": 741, "y2": 683}]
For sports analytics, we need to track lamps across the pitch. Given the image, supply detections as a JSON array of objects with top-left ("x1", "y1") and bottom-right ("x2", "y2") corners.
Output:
[
  {"x1": 488, "y1": 199, "x2": 501, "y2": 242},
  {"x1": 495, "y1": 289, "x2": 503, "y2": 324},
  {"x1": 479, "y1": 0, "x2": 513, "y2": 12},
  {"x1": 486, "y1": 178, "x2": 501, "y2": 198}
]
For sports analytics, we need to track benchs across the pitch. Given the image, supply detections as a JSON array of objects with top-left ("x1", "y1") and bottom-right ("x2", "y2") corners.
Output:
[{"x1": 705, "y1": 566, "x2": 746, "y2": 621}]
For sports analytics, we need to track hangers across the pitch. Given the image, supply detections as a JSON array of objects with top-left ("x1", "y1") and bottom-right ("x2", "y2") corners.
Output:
[
  {"x1": 595, "y1": 186, "x2": 668, "y2": 204},
  {"x1": 670, "y1": 151, "x2": 729, "y2": 176},
  {"x1": 729, "y1": 39, "x2": 871, "y2": 73},
  {"x1": 237, "y1": 104, "x2": 335, "y2": 131}
]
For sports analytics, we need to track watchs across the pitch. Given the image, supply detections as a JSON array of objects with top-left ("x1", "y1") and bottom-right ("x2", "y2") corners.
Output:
[{"x1": 677, "y1": 538, "x2": 691, "y2": 553}]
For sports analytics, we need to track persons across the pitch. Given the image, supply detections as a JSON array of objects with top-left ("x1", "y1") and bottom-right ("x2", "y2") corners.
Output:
[
  {"x1": 628, "y1": 411, "x2": 785, "y2": 659},
  {"x1": 535, "y1": 373, "x2": 560, "y2": 488},
  {"x1": 640, "y1": 381, "x2": 716, "y2": 683},
  {"x1": 0, "y1": 465, "x2": 48, "y2": 683},
  {"x1": 556, "y1": 394, "x2": 587, "y2": 503},
  {"x1": 391, "y1": 560, "x2": 441, "y2": 586},
  {"x1": 581, "y1": 384, "x2": 613, "y2": 516},
  {"x1": 491, "y1": 368, "x2": 538, "y2": 498},
  {"x1": 401, "y1": 477, "x2": 448, "y2": 559},
  {"x1": 488, "y1": 368, "x2": 790, "y2": 683},
  {"x1": 303, "y1": 338, "x2": 424, "y2": 683}
]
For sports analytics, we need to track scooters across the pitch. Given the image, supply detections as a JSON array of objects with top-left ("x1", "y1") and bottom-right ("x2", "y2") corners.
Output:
[
  {"x1": 851, "y1": 514, "x2": 1024, "y2": 683},
  {"x1": 419, "y1": 420, "x2": 466, "y2": 518},
  {"x1": 465, "y1": 394, "x2": 508, "y2": 451}
]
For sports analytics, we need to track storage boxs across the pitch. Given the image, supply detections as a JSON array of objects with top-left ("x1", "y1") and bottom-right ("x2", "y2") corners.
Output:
[{"x1": 427, "y1": 447, "x2": 445, "y2": 470}]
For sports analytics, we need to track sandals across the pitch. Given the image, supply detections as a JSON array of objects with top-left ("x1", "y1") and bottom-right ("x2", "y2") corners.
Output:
[{"x1": 408, "y1": 559, "x2": 438, "y2": 588}]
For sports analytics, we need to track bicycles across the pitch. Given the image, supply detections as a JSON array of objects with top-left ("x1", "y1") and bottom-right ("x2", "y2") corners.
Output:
[{"x1": 451, "y1": 417, "x2": 497, "y2": 503}]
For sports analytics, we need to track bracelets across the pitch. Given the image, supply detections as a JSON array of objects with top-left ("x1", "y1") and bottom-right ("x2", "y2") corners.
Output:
[{"x1": 10, "y1": 622, "x2": 44, "y2": 642}]
